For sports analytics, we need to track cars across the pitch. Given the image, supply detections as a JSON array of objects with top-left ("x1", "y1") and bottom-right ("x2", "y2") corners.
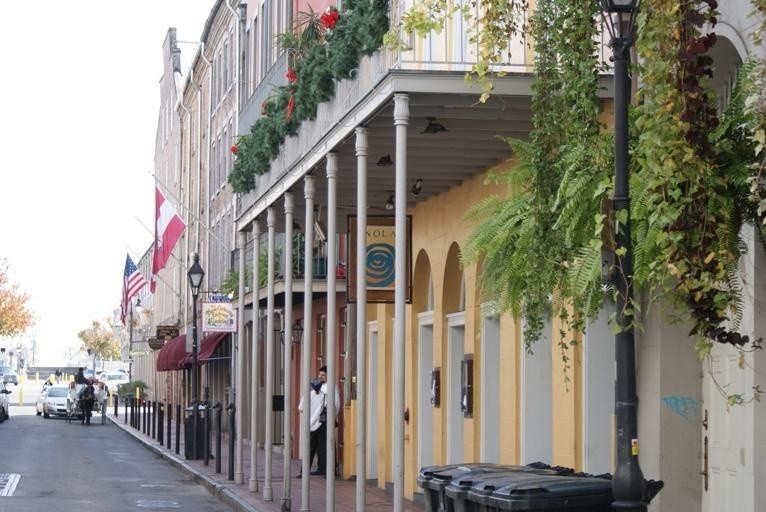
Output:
[
  {"x1": 0, "y1": 366, "x2": 18, "y2": 426},
  {"x1": 35, "y1": 364, "x2": 130, "y2": 419}
]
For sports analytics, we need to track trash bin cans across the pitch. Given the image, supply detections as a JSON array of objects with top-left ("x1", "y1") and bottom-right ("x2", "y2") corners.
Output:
[
  {"x1": 183, "y1": 404, "x2": 214, "y2": 460},
  {"x1": 416, "y1": 460, "x2": 664, "y2": 512}
]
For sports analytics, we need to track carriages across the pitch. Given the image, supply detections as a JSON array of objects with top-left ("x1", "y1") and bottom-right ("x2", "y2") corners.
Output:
[{"x1": 64, "y1": 384, "x2": 108, "y2": 425}]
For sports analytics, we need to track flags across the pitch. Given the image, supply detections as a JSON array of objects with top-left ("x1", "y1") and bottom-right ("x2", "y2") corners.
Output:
[
  {"x1": 121, "y1": 252, "x2": 150, "y2": 323},
  {"x1": 150, "y1": 186, "x2": 187, "y2": 294}
]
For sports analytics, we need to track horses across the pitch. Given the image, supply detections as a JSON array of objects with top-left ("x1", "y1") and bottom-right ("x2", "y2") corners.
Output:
[{"x1": 79, "y1": 381, "x2": 95, "y2": 425}]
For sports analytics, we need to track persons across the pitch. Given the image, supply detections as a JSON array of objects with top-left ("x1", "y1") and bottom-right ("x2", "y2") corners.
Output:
[
  {"x1": 297, "y1": 380, "x2": 327, "y2": 478},
  {"x1": 55, "y1": 369, "x2": 62, "y2": 384},
  {"x1": 78, "y1": 368, "x2": 88, "y2": 383},
  {"x1": 311, "y1": 366, "x2": 341, "y2": 476}
]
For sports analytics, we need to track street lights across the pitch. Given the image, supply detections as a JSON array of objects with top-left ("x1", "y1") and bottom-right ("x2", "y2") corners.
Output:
[
  {"x1": 187, "y1": 252, "x2": 206, "y2": 463},
  {"x1": 594, "y1": 0, "x2": 647, "y2": 512}
]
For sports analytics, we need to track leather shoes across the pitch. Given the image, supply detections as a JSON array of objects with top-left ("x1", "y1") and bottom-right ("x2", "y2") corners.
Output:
[
  {"x1": 295, "y1": 473, "x2": 302, "y2": 478},
  {"x1": 310, "y1": 469, "x2": 320, "y2": 475}
]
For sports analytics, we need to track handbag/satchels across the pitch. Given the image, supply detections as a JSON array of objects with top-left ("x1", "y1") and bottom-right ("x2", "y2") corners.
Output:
[{"x1": 319, "y1": 394, "x2": 338, "y2": 427}]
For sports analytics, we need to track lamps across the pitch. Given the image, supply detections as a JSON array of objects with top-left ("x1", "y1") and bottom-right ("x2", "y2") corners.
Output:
[
  {"x1": 291, "y1": 320, "x2": 303, "y2": 343},
  {"x1": 376, "y1": 154, "x2": 393, "y2": 166},
  {"x1": 420, "y1": 117, "x2": 448, "y2": 134},
  {"x1": 385, "y1": 195, "x2": 394, "y2": 210},
  {"x1": 411, "y1": 180, "x2": 423, "y2": 196}
]
